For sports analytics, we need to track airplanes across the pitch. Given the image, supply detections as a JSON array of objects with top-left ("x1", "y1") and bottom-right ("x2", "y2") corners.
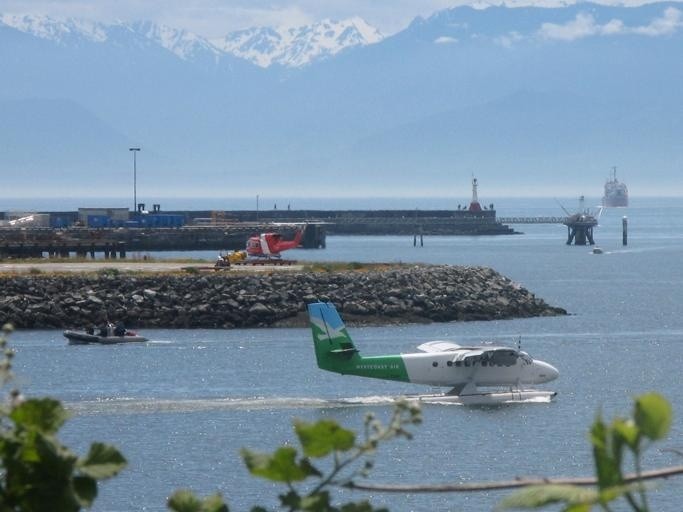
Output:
[{"x1": 307, "y1": 301, "x2": 560, "y2": 406}]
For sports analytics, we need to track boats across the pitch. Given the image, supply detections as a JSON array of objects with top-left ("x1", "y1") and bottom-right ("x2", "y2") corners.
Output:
[{"x1": 62, "y1": 327, "x2": 148, "y2": 345}]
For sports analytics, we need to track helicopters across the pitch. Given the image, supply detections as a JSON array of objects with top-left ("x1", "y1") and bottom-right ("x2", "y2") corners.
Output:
[{"x1": 245, "y1": 219, "x2": 337, "y2": 259}]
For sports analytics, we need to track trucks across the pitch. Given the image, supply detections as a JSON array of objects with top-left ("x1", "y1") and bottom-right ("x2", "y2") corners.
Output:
[{"x1": 228, "y1": 249, "x2": 297, "y2": 266}]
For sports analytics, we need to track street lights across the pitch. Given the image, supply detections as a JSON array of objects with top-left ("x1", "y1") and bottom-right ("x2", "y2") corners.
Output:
[{"x1": 130, "y1": 147, "x2": 141, "y2": 218}]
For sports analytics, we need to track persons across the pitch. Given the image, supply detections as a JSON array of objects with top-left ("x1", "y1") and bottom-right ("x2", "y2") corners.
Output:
[
  {"x1": 463, "y1": 205, "x2": 467, "y2": 210},
  {"x1": 471, "y1": 172, "x2": 479, "y2": 202},
  {"x1": 484, "y1": 203, "x2": 493, "y2": 210},
  {"x1": 85, "y1": 319, "x2": 126, "y2": 337},
  {"x1": 458, "y1": 203, "x2": 461, "y2": 210}
]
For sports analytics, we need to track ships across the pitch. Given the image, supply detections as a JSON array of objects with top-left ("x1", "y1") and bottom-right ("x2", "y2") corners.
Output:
[{"x1": 603, "y1": 166, "x2": 628, "y2": 208}]
